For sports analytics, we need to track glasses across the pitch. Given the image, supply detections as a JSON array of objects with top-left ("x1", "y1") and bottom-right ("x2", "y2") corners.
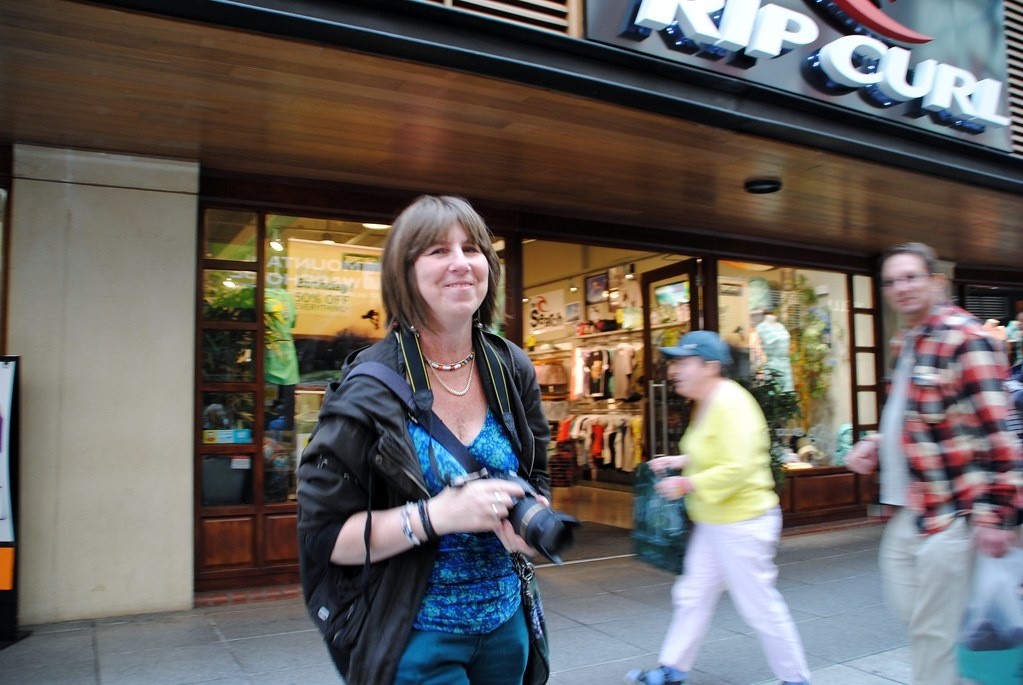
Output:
[{"x1": 882, "y1": 272, "x2": 930, "y2": 285}]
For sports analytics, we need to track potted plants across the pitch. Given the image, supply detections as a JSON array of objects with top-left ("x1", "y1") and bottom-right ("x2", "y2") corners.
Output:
[{"x1": 197, "y1": 286, "x2": 297, "y2": 502}]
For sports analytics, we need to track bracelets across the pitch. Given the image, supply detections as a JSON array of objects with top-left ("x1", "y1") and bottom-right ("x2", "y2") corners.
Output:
[{"x1": 402, "y1": 500, "x2": 439, "y2": 546}]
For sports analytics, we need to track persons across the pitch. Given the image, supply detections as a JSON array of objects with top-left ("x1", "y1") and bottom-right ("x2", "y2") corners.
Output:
[
  {"x1": 756, "y1": 315, "x2": 796, "y2": 397},
  {"x1": 846, "y1": 243, "x2": 1022, "y2": 685},
  {"x1": 625, "y1": 329, "x2": 811, "y2": 685},
  {"x1": 295, "y1": 194, "x2": 552, "y2": 684}
]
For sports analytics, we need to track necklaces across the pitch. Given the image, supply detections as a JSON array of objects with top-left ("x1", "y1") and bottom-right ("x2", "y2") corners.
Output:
[{"x1": 422, "y1": 348, "x2": 476, "y2": 396}]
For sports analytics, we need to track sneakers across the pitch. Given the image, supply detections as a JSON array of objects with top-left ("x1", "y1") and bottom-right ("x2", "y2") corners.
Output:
[{"x1": 623, "y1": 665, "x2": 687, "y2": 684}]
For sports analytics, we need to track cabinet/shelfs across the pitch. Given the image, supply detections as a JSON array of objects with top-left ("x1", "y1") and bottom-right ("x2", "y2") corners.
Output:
[{"x1": 526, "y1": 320, "x2": 689, "y2": 530}]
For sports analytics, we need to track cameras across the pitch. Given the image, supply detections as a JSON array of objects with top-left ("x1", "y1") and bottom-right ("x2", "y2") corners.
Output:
[{"x1": 492, "y1": 470, "x2": 583, "y2": 567}]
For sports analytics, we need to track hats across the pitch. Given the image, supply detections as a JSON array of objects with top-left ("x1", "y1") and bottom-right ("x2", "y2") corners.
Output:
[{"x1": 658, "y1": 330, "x2": 733, "y2": 366}]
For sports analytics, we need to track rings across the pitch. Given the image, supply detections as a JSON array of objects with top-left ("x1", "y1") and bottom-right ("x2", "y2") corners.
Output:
[
  {"x1": 490, "y1": 504, "x2": 499, "y2": 518},
  {"x1": 495, "y1": 492, "x2": 503, "y2": 503}
]
